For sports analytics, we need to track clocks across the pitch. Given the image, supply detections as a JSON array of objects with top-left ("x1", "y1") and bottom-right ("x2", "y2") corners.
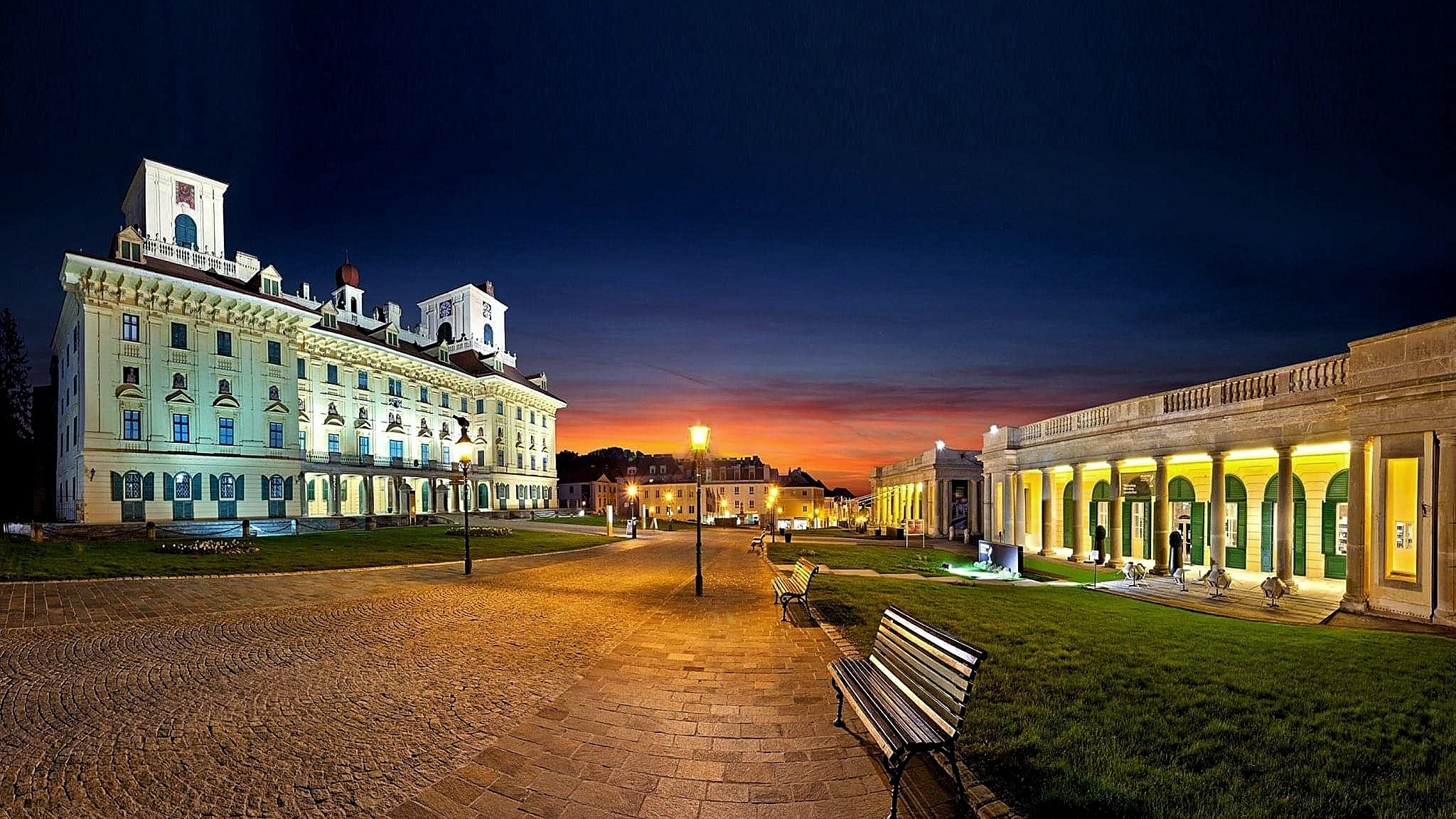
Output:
[
  {"x1": 440, "y1": 302, "x2": 452, "y2": 317},
  {"x1": 483, "y1": 303, "x2": 491, "y2": 318}
]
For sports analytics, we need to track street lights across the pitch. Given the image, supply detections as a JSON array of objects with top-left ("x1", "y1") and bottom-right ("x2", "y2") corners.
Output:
[
  {"x1": 768, "y1": 496, "x2": 774, "y2": 535},
  {"x1": 687, "y1": 421, "x2": 711, "y2": 598},
  {"x1": 777, "y1": 507, "x2": 783, "y2": 519},
  {"x1": 454, "y1": 428, "x2": 475, "y2": 576},
  {"x1": 770, "y1": 488, "x2": 778, "y2": 544},
  {"x1": 766, "y1": 502, "x2": 772, "y2": 531},
  {"x1": 721, "y1": 499, "x2": 727, "y2": 530},
  {"x1": 627, "y1": 484, "x2": 639, "y2": 539},
  {"x1": 665, "y1": 493, "x2": 673, "y2": 530}
]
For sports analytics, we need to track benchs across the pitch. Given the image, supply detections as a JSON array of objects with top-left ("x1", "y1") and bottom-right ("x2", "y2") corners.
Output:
[
  {"x1": 826, "y1": 604, "x2": 989, "y2": 819},
  {"x1": 751, "y1": 530, "x2": 765, "y2": 552},
  {"x1": 773, "y1": 557, "x2": 819, "y2": 622}
]
